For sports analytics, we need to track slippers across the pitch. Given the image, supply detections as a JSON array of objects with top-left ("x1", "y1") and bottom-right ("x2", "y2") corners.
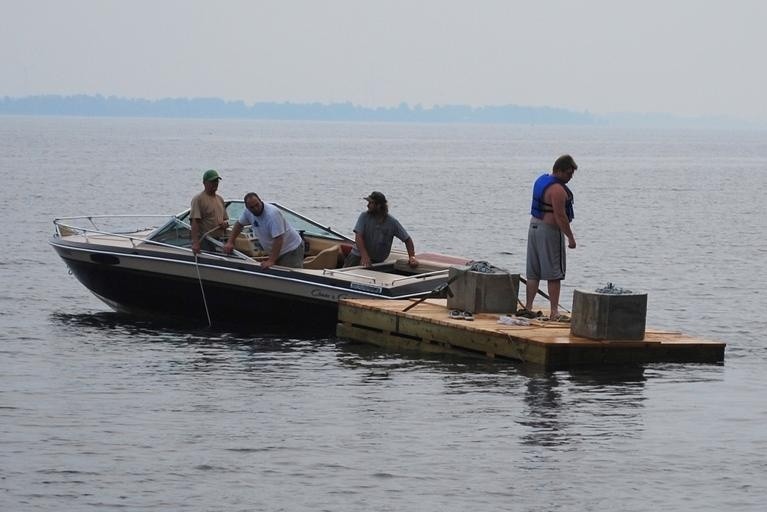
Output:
[{"x1": 449, "y1": 310, "x2": 474, "y2": 320}]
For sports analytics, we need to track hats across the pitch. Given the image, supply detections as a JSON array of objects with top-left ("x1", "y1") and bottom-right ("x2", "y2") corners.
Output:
[
  {"x1": 363, "y1": 192, "x2": 386, "y2": 204},
  {"x1": 203, "y1": 170, "x2": 222, "y2": 182}
]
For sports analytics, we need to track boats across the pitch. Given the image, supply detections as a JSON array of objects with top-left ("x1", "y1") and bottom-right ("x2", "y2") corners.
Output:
[{"x1": 46, "y1": 207, "x2": 503, "y2": 337}]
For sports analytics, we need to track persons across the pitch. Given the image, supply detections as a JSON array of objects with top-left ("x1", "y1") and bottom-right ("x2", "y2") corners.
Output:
[
  {"x1": 188, "y1": 168, "x2": 230, "y2": 255},
  {"x1": 522, "y1": 154, "x2": 576, "y2": 321},
  {"x1": 341, "y1": 191, "x2": 419, "y2": 271},
  {"x1": 223, "y1": 191, "x2": 305, "y2": 271}
]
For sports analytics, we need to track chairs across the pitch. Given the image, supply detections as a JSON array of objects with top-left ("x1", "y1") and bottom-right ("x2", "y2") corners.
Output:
[
  {"x1": 234, "y1": 236, "x2": 265, "y2": 257},
  {"x1": 302, "y1": 244, "x2": 339, "y2": 269}
]
